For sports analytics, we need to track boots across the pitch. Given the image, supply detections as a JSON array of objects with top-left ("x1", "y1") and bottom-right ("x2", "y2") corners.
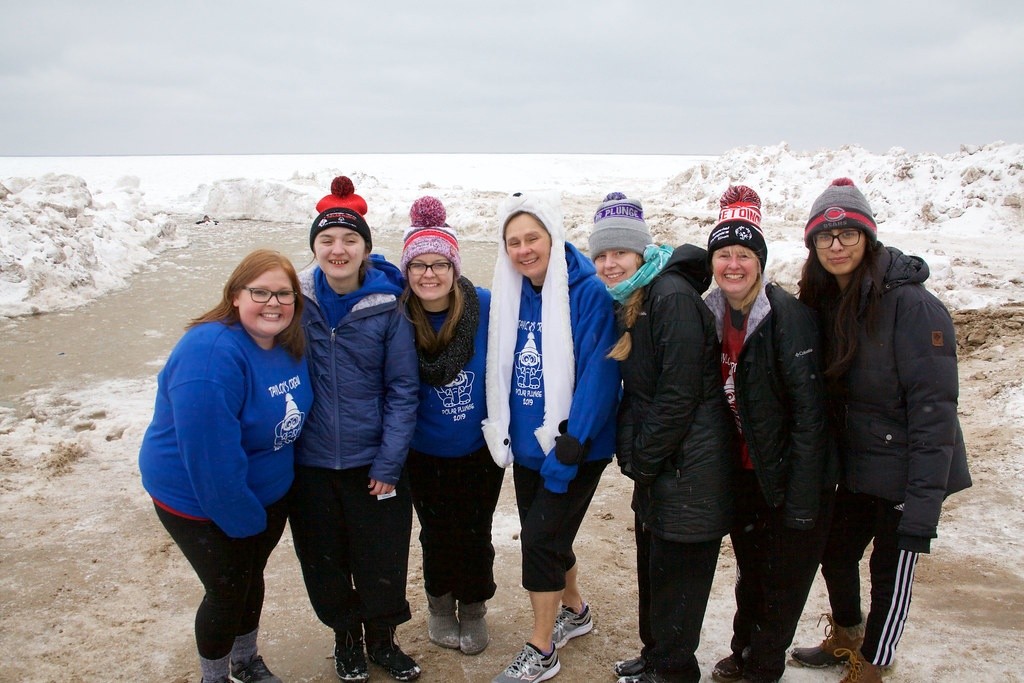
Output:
[
  {"x1": 792, "y1": 613, "x2": 866, "y2": 667},
  {"x1": 457, "y1": 602, "x2": 489, "y2": 655},
  {"x1": 424, "y1": 591, "x2": 460, "y2": 648},
  {"x1": 334, "y1": 625, "x2": 369, "y2": 681},
  {"x1": 833, "y1": 647, "x2": 897, "y2": 683},
  {"x1": 364, "y1": 625, "x2": 420, "y2": 679}
]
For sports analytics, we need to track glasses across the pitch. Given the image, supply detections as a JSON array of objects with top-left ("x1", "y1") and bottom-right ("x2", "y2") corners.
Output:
[
  {"x1": 406, "y1": 262, "x2": 454, "y2": 275},
  {"x1": 240, "y1": 286, "x2": 298, "y2": 305},
  {"x1": 812, "y1": 230, "x2": 862, "y2": 249}
]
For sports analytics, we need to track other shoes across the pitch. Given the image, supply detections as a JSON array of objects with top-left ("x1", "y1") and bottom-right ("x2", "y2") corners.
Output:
[{"x1": 712, "y1": 653, "x2": 746, "y2": 682}]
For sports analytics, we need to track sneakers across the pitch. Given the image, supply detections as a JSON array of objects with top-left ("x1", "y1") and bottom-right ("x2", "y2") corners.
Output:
[
  {"x1": 615, "y1": 655, "x2": 648, "y2": 673},
  {"x1": 618, "y1": 669, "x2": 672, "y2": 683},
  {"x1": 550, "y1": 604, "x2": 592, "y2": 649},
  {"x1": 492, "y1": 642, "x2": 561, "y2": 683},
  {"x1": 230, "y1": 656, "x2": 282, "y2": 683}
]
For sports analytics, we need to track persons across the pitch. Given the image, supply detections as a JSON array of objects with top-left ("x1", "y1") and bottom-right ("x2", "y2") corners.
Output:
[
  {"x1": 791, "y1": 177, "x2": 970, "y2": 683},
  {"x1": 586, "y1": 191, "x2": 740, "y2": 683},
  {"x1": 285, "y1": 177, "x2": 421, "y2": 683},
  {"x1": 400, "y1": 197, "x2": 507, "y2": 654},
  {"x1": 483, "y1": 187, "x2": 620, "y2": 683},
  {"x1": 137, "y1": 252, "x2": 315, "y2": 683},
  {"x1": 701, "y1": 187, "x2": 822, "y2": 683}
]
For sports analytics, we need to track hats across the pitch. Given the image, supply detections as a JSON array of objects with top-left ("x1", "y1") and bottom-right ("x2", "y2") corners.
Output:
[
  {"x1": 480, "y1": 191, "x2": 574, "y2": 468},
  {"x1": 708, "y1": 185, "x2": 767, "y2": 272},
  {"x1": 589, "y1": 193, "x2": 651, "y2": 265},
  {"x1": 310, "y1": 176, "x2": 372, "y2": 244},
  {"x1": 804, "y1": 178, "x2": 878, "y2": 249},
  {"x1": 400, "y1": 196, "x2": 461, "y2": 279}
]
